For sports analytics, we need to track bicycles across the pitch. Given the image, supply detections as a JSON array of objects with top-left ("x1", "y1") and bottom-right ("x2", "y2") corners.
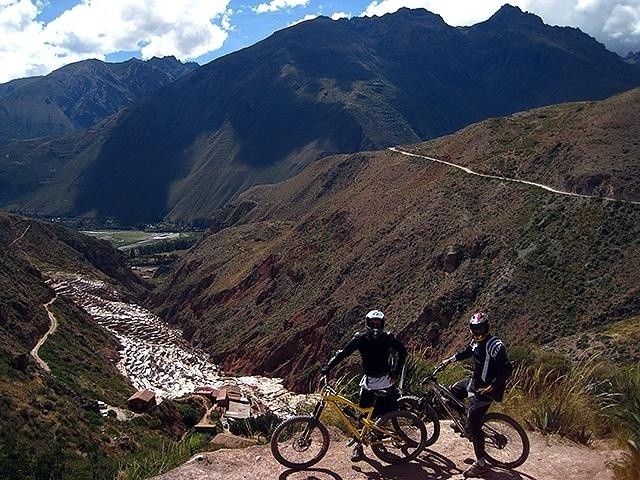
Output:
[{"x1": 272, "y1": 365, "x2": 529, "y2": 469}]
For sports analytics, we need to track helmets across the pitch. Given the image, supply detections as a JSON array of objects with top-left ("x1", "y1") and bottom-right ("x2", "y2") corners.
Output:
[
  {"x1": 365, "y1": 310, "x2": 385, "y2": 336},
  {"x1": 468, "y1": 313, "x2": 489, "y2": 342}
]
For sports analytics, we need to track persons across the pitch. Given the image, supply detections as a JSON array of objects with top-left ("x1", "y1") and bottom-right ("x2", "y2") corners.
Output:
[
  {"x1": 440, "y1": 313, "x2": 514, "y2": 477},
  {"x1": 321, "y1": 310, "x2": 408, "y2": 462}
]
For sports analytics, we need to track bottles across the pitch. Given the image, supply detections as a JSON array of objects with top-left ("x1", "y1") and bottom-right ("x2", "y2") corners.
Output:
[{"x1": 341, "y1": 405, "x2": 363, "y2": 423}]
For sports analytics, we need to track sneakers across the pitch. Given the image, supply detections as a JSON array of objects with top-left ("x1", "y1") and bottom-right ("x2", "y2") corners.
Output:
[
  {"x1": 450, "y1": 418, "x2": 466, "y2": 429},
  {"x1": 377, "y1": 443, "x2": 387, "y2": 453},
  {"x1": 463, "y1": 462, "x2": 489, "y2": 477},
  {"x1": 351, "y1": 445, "x2": 363, "y2": 461}
]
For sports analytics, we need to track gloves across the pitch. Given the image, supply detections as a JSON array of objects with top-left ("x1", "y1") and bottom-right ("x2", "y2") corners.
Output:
[
  {"x1": 387, "y1": 368, "x2": 400, "y2": 384},
  {"x1": 320, "y1": 365, "x2": 330, "y2": 378}
]
